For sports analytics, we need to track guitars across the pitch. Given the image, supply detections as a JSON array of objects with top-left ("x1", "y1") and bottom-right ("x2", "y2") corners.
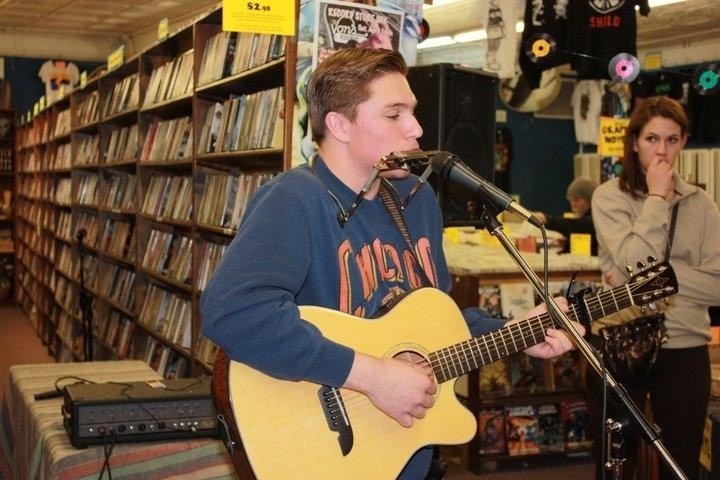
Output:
[{"x1": 211, "y1": 255, "x2": 678, "y2": 480}]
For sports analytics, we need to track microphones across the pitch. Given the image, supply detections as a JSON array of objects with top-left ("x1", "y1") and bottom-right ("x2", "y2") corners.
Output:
[{"x1": 432, "y1": 151, "x2": 544, "y2": 228}]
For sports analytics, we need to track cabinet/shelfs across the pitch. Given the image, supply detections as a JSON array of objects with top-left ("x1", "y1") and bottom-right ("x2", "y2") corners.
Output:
[
  {"x1": 1, "y1": 0, "x2": 413, "y2": 386},
  {"x1": 442, "y1": 267, "x2": 650, "y2": 480}
]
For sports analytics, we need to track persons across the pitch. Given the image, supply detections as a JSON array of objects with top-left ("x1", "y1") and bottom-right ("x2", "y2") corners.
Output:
[
  {"x1": 199, "y1": 48, "x2": 587, "y2": 480},
  {"x1": 533, "y1": 179, "x2": 600, "y2": 255},
  {"x1": 591, "y1": 94, "x2": 720, "y2": 480}
]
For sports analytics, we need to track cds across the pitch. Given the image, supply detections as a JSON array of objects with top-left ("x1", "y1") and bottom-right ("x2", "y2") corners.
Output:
[
  {"x1": 525, "y1": 33, "x2": 558, "y2": 66},
  {"x1": 692, "y1": 64, "x2": 720, "y2": 96},
  {"x1": 608, "y1": 53, "x2": 640, "y2": 83}
]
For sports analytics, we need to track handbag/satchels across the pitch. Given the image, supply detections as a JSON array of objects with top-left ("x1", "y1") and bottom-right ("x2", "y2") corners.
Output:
[{"x1": 599, "y1": 313, "x2": 668, "y2": 381}]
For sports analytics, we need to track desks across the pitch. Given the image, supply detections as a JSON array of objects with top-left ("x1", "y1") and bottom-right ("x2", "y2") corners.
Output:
[{"x1": 5, "y1": 360, "x2": 240, "y2": 479}]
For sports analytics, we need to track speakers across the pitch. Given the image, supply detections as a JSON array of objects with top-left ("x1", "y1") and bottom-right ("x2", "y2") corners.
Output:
[{"x1": 407, "y1": 63, "x2": 498, "y2": 229}]
